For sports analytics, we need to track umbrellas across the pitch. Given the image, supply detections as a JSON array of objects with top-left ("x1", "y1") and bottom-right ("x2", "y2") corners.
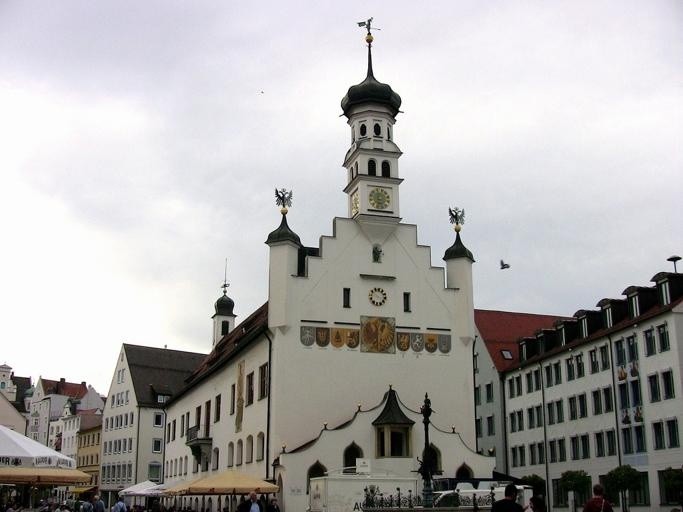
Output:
[
  {"x1": 0, "y1": 424, "x2": 77, "y2": 469},
  {"x1": 118, "y1": 465, "x2": 280, "y2": 494},
  {"x1": 0, "y1": 469, "x2": 92, "y2": 485}
]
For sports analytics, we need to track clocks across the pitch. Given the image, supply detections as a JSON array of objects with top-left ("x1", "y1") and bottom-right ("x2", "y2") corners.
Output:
[
  {"x1": 369, "y1": 288, "x2": 386, "y2": 306},
  {"x1": 369, "y1": 188, "x2": 390, "y2": 209},
  {"x1": 351, "y1": 192, "x2": 360, "y2": 216}
]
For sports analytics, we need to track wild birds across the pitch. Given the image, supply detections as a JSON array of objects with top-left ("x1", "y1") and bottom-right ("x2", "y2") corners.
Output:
[{"x1": 500, "y1": 260, "x2": 510, "y2": 269}]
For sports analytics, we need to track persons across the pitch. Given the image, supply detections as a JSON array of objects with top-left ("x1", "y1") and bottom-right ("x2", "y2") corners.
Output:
[
  {"x1": 265, "y1": 498, "x2": 280, "y2": 512},
  {"x1": 491, "y1": 484, "x2": 523, "y2": 512},
  {"x1": 582, "y1": 485, "x2": 613, "y2": 512},
  {"x1": 0, "y1": 486, "x2": 127, "y2": 512},
  {"x1": 238, "y1": 491, "x2": 263, "y2": 512}
]
